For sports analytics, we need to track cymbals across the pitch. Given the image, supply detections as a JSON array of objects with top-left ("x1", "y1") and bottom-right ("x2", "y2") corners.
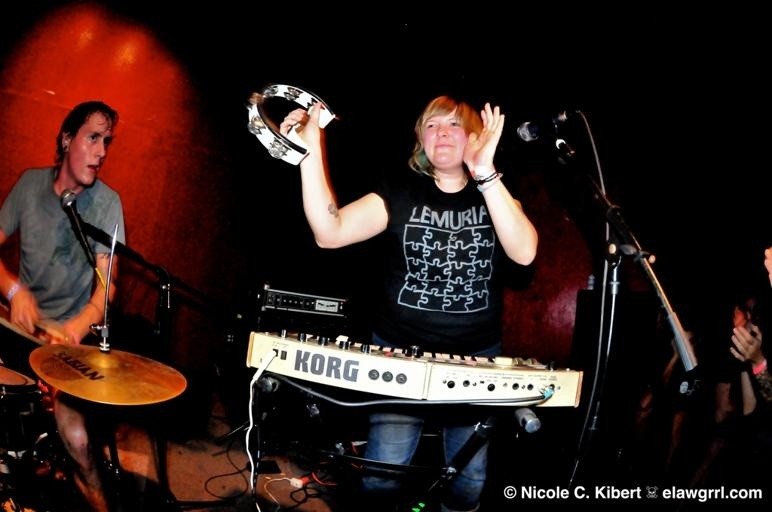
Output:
[{"x1": 30, "y1": 344, "x2": 187, "y2": 407}]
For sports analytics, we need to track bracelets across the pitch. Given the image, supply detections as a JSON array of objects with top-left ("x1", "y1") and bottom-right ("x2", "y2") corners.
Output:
[
  {"x1": 751, "y1": 356, "x2": 769, "y2": 378},
  {"x1": 472, "y1": 168, "x2": 504, "y2": 192},
  {"x1": 4, "y1": 280, "x2": 26, "y2": 302}
]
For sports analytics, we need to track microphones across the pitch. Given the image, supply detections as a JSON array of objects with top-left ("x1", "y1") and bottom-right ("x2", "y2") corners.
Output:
[
  {"x1": 517, "y1": 111, "x2": 566, "y2": 142},
  {"x1": 59, "y1": 190, "x2": 98, "y2": 271}
]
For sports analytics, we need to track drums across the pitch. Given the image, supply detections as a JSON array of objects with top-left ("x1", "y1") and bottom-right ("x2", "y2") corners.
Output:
[{"x1": 0, "y1": 366, "x2": 35, "y2": 448}]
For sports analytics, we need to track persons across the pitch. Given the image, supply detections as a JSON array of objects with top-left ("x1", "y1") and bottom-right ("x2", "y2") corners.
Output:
[
  {"x1": 664, "y1": 330, "x2": 731, "y2": 451},
  {"x1": 727, "y1": 322, "x2": 770, "y2": 410},
  {"x1": 762, "y1": 248, "x2": 772, "y2": 285},
  {"x1": 278, "y1": 94, "x2": 539, "y2": 512},
  {"x1": 1, "y1": 101, "x2": 129, "y2": 512}
]
